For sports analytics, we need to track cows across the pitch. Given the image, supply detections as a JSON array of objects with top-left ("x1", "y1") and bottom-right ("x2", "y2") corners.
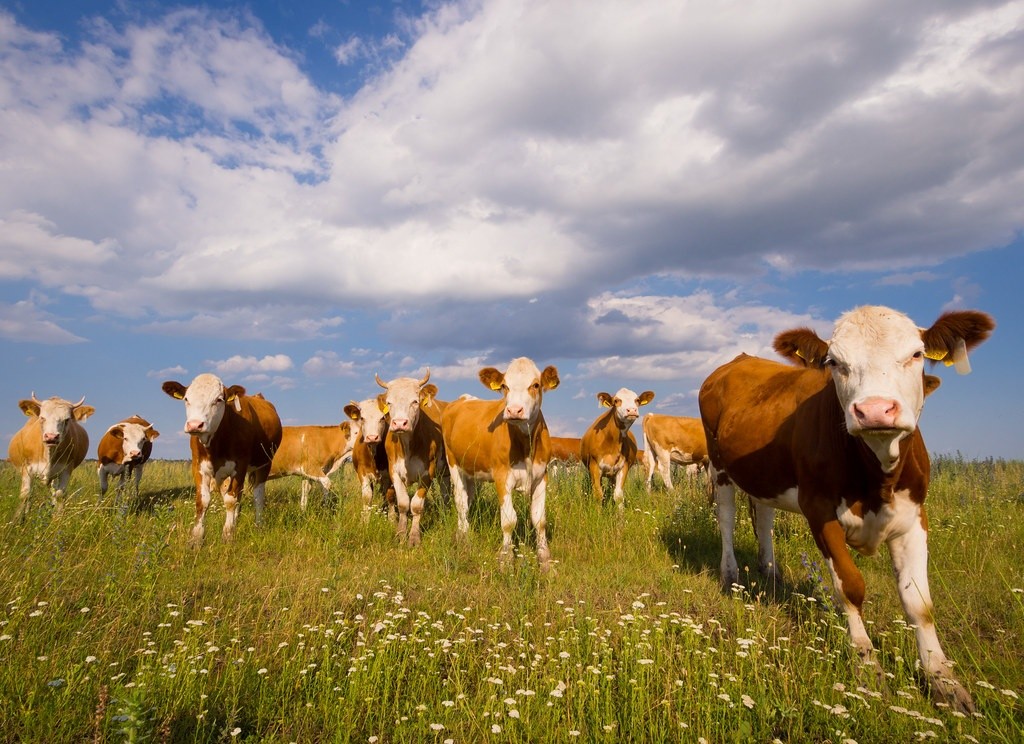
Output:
[
  {"x1": 8, "y1": 391, "x2": 96, "y2": 522},
  {"x1": 161, "y1": 373, "x2": 283, "y2": 550},
  {"x1": 441, "y1": 358, "x2": 561, "y2": 578},
  {"x1": 548, "y1": 436, "x2": 586, "y2": 477},
  {"x1": 579, "y1": 387, "x2": 654, "y2": 513},
  {"x1": 642, "y1": 411, "x2": 713, "y2": 492},
  {"x1": 273, "y1": 421, "x2": 361, "y2": 516},
  {"x1": 698, "y1": 303, "x2": 996, "y2": 713},
  {"x1": 96, "y1": 414, "x2": 160, "y2": 509},
  {"x1": 339, "y1": 366, "x2": 448, "y2": 552}
]
